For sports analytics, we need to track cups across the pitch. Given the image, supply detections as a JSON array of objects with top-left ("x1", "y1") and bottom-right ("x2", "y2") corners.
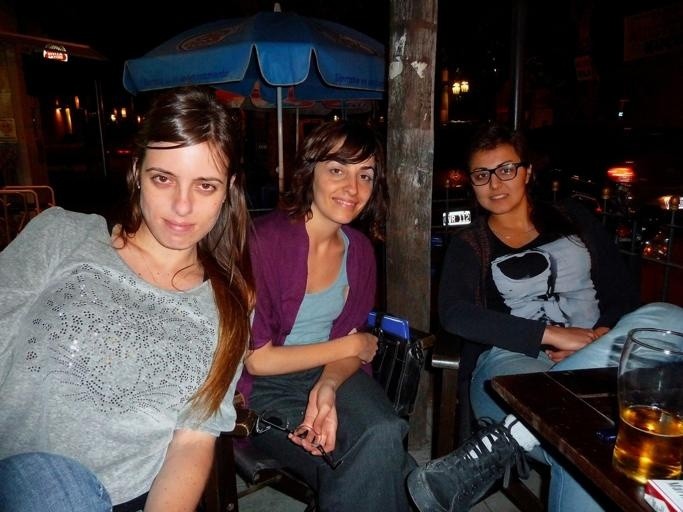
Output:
[{"x1": 612, "y1": 328, "x2": 682, "y2": 483}]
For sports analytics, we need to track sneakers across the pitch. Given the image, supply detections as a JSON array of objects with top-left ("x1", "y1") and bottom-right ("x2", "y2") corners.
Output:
[{"x1": 407, "y1": 423, "x2": 527, "y2": 512}]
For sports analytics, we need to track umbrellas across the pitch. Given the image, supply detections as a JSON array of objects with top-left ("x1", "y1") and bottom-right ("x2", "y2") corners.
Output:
[
  {"x1": 121, "y1": 2, "x2": 389, "y2": 203},
  {"x1": 213, "y1": 81, "x2": 372, "y2": 165}
]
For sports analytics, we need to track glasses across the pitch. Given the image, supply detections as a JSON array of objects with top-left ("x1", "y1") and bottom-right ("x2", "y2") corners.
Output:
[
  {"x1": 259, "y1": 408, "x2": 343, "y2": 472},
  {"x1": 468, "y1": 162, "x2": 524, "y2": 185}
]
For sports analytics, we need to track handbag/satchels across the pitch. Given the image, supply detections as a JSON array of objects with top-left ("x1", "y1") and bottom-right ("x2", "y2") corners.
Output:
[{"x1": 355, "y1": 311, "x2": 425, "y2": 419}]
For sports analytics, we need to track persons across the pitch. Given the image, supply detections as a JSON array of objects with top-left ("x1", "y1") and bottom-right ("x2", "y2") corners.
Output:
[
  {"x1": 0, "y1": 89, "x2": 258, "y2": 512},
  {"x1": 405, "y1": 123, "x2": 682, "y2": 511},
  {"x1": 217, "y1": 117, "x2": 419, "y2": 511}
]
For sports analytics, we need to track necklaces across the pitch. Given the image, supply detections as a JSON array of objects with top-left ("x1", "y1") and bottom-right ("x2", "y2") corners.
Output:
[{"x1": 491, "y1": 215, "x2": 537, "y2": 239}]
